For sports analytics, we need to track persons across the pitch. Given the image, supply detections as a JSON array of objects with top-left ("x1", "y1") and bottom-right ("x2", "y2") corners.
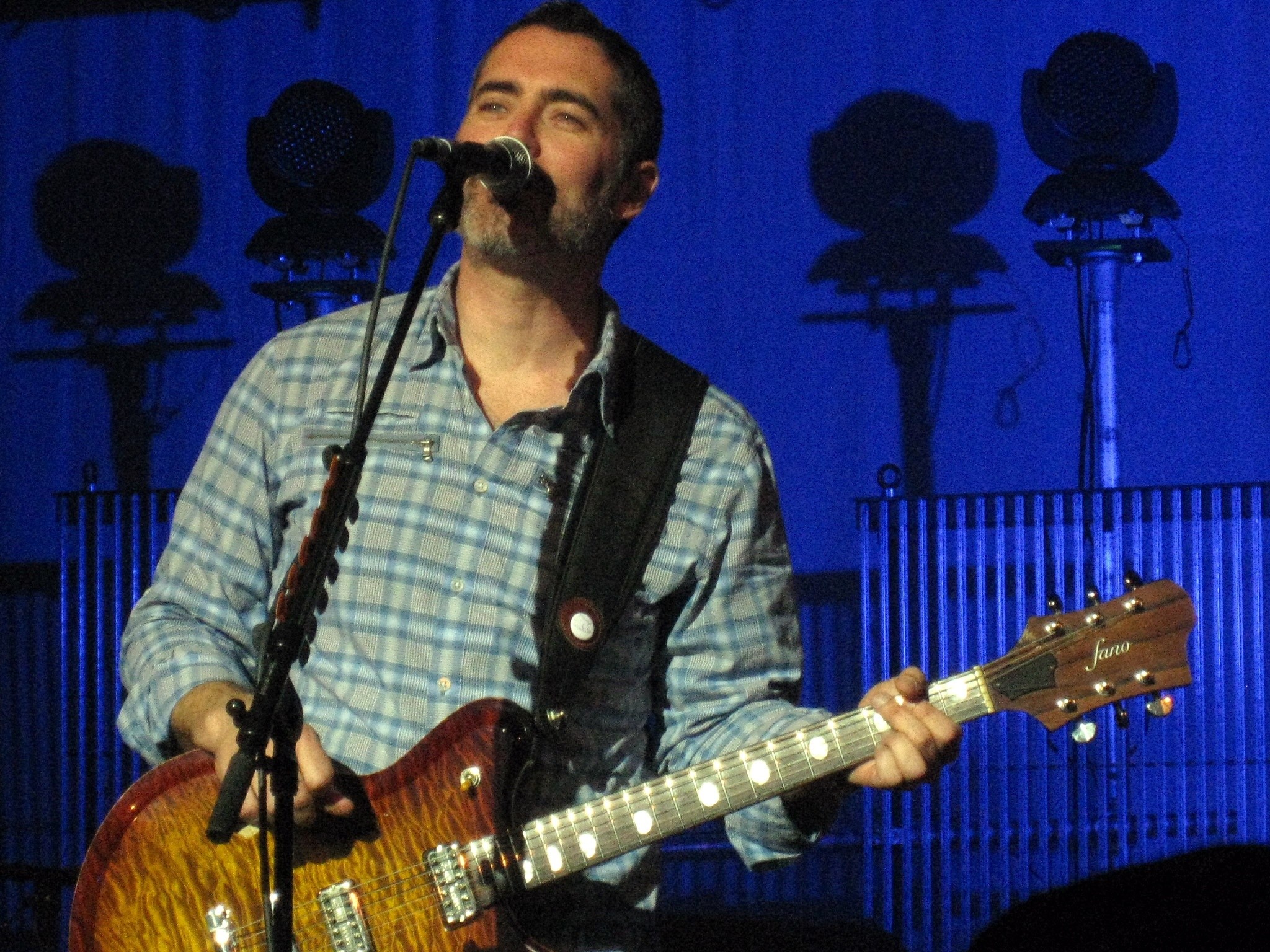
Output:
[{"x1": 115, "y1": 0, "x2": 960, "y2": 952}]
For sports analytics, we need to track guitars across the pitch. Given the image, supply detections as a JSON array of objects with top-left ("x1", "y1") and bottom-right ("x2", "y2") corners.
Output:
[{"x1": 68, "y1": 570, "x2": 1197, "y2": 952}]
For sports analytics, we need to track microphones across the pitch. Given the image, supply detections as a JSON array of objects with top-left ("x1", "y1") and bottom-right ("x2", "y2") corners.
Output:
[{"x1": 412, "y1": 135, "x2": 532, "y2": 197}]
